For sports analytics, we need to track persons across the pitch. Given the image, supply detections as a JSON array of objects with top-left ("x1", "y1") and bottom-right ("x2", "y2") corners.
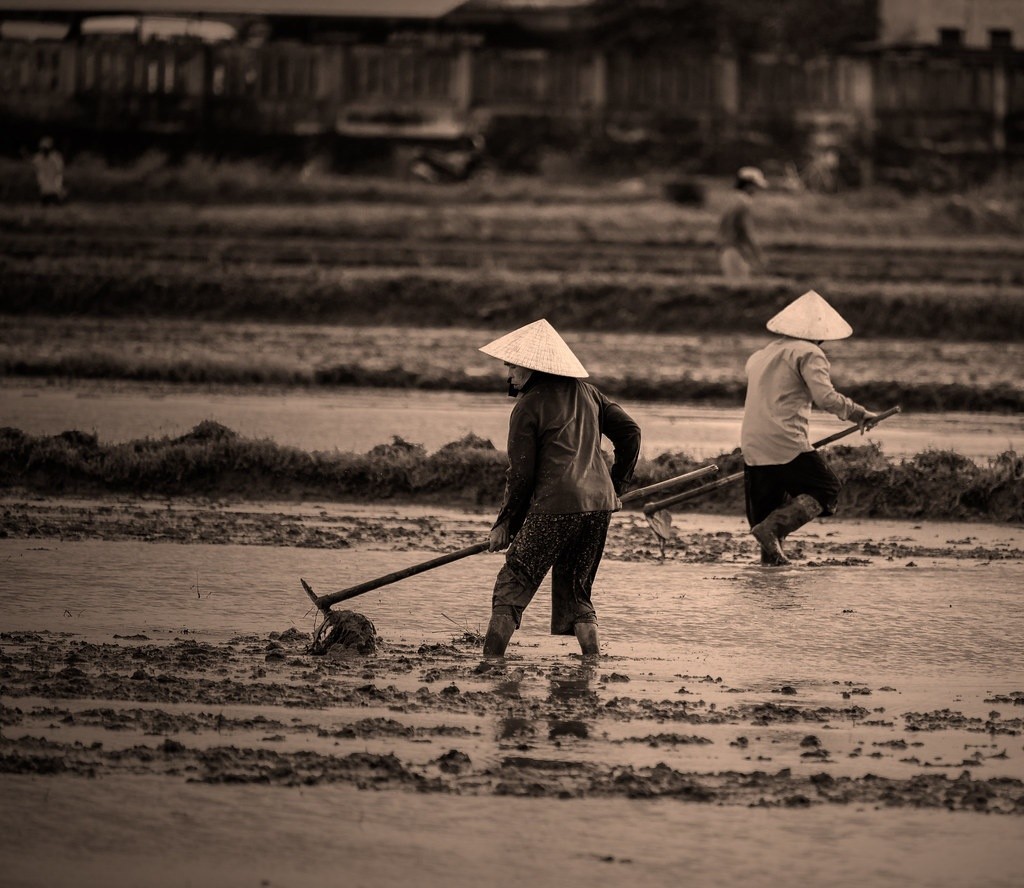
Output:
[
  {"x1": 477, "y1": 319, "x2": 641, "y2": 672},
  {"x1": 717, "y1": 166, "x2": 770, "y2": 277},
  {"x1": 739, "y1": 289, "x2": 878, "y2": 567},
  {"x1": 30, "y1": 139, "x2": 64, "y2": 205}
]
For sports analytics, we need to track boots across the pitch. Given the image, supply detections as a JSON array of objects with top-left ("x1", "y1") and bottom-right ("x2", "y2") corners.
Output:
[{"x1": 752, "y1": 493, "x2": 823, "y2": 566}]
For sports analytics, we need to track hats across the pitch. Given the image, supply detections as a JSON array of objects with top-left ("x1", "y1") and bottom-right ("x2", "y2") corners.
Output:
[
  {"x1": 765, "y1": 289, "x2": 854, "y2": 341},
  {"x1": 478, "y1": 318, "x2": 590, "y2": 379},
  {"x1": 39, "y1": 138, "x2": 53, "y2": 149},
  {"x1": 738, "y1": 165, "x2": 770, "y2": 189}
]
